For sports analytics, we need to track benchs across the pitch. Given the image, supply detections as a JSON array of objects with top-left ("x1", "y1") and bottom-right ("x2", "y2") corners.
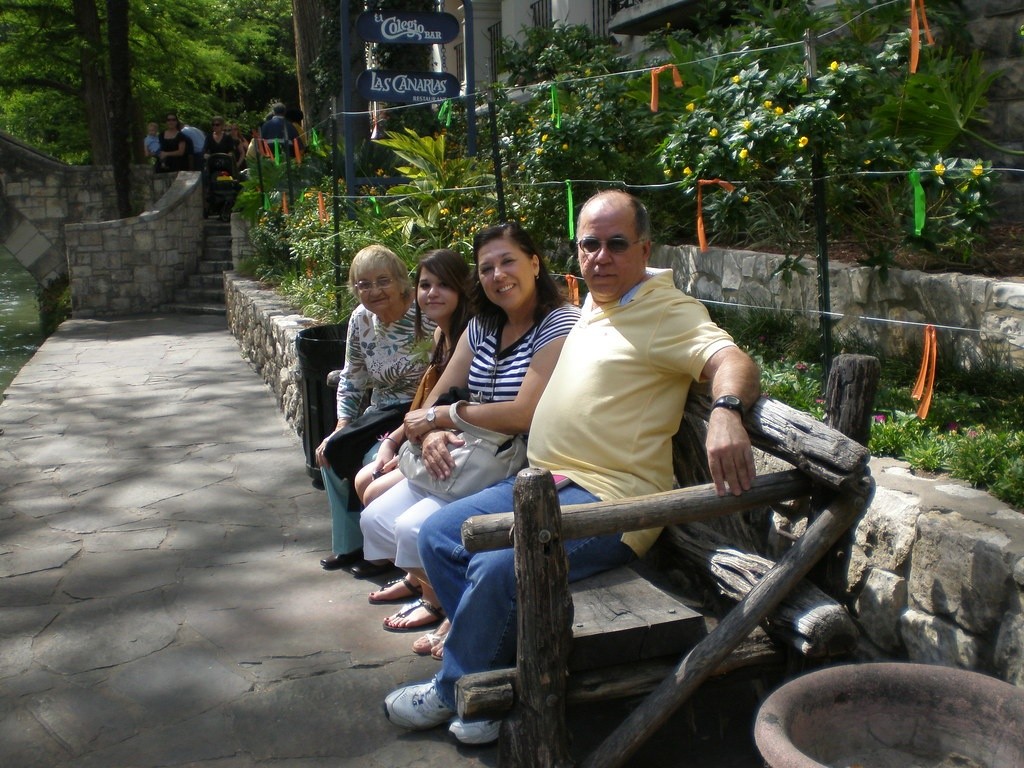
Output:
[{"x1": 327, "y1": 354, "x2": 879, "y2": 768}]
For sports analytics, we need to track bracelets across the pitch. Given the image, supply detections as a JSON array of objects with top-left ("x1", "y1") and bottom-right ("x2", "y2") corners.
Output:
[{"x1": 378, "y1": 433, "x2": 398, "y2": 448}]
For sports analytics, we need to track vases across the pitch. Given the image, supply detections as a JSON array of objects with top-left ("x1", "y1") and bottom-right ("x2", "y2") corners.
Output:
[{"x1": 755, "y1": 662, "x2": 1024, "y2": 768}]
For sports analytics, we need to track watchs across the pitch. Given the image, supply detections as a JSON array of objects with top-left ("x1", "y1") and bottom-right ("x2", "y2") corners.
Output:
[
  {"x1": 711, "y1": 395, "x2": 744, "y2": 424},
  {"x1": 426, "y1": 406, "x2": 437, "y2": 430}
]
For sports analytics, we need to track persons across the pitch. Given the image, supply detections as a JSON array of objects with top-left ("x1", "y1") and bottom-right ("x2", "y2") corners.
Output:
[
  {"x1": 361, "y1": 222, "x2": 581, "y2": 661},
  {"x1": 144, "y1": 102, "x2": 304, "y2": 208},
  {"x1": 383, "y1": 191, "x2": 761, "y2": 744},
  {"x1": 354, "y1": 250, "x2": 473, "y2": 632},
  {"x1": 316, "y1": 245, "x2": 440, "y2": 576}
]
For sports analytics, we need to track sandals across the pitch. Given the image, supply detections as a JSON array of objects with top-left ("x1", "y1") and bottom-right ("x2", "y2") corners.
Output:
[
  {"x1": 431, "y1": 635, "x2": 449, "y2": 660},
  {"x1": 412, "y1": 631, "x2": 443, "y2": 654},
  {"x1": 384, "y1": 597, "x2": 444, "y2": 632},
  {"x1": 368, "y1": 576, "x2": 422, "y2": 603}
]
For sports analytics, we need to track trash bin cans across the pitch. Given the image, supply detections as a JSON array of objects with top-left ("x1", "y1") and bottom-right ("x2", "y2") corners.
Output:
[{"x1": 296, "y1": 323, "x2": 373, "y2": 480}]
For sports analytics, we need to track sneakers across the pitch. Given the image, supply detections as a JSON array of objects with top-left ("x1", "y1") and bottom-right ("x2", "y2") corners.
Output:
[
  {"x1": 383, "y1": 673, "x2": 455, "y2": 731},
  {"x1": 450, "y1": 718, "x2": 503, "y2": 745}
]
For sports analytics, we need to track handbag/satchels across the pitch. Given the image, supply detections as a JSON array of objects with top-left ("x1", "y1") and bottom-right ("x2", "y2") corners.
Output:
[{"x1": 397, "y1": 399, "x2": 527, "y2": 503}]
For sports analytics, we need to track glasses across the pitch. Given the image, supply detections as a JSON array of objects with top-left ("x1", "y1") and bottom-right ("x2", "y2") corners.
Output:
[
  {"x1": 575, "y1": 238, "x2": 645, "y2": 253},
  {"x1": 166, "y1": 118, "x2": 176, "y2": 122},
  {"x1": 212, "y1": 123, "x2": 221, "y2": 126},
  {"x1": 355, "y1": 277, "x2": 396, "y2": 290}
]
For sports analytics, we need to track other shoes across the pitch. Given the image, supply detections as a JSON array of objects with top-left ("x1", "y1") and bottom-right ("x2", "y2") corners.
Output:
[
  {"x1": 349, "y1": 557, "x2": 393, "y2": 575},
  {"x1": 320, "y1": 550, "x2": 362, "y2": 568}
]
